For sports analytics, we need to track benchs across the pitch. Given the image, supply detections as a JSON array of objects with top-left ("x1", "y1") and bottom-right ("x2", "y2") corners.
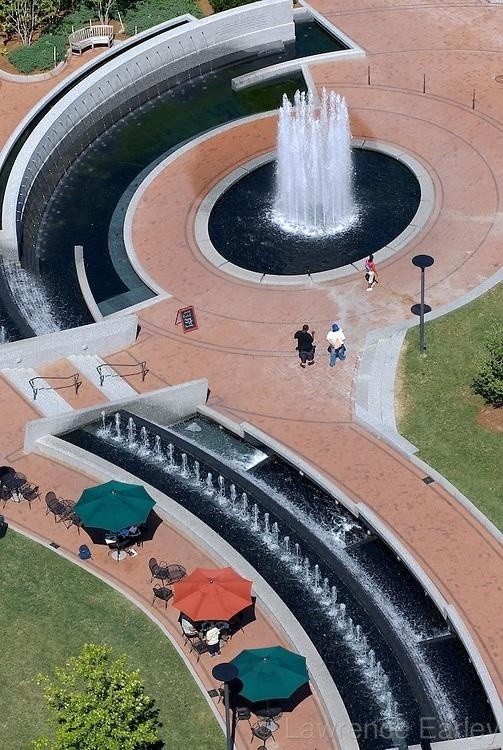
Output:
[{"x1": 67, "y1": 24, "x2": 114, "y2": 56}]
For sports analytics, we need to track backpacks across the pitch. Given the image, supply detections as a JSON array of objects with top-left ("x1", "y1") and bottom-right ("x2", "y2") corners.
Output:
[{"x1": 79, "y1": 544, "x2": 92, "y2": 560}]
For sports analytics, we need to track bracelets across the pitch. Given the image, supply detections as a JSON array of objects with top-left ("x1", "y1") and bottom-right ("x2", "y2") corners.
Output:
[{"x1": 375, "y1": 272, "x2": 377, "y2": 274}]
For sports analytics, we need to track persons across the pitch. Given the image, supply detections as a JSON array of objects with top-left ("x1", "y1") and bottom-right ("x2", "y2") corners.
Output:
[
  {"x1": 363, "y1": 253, "x2": 380, "y2": 279},
  {"x1": 292, "y1": 323, "x2": 317, "y2": 369},
  {"x1": 180, "y1": 614, "x2": 241, "y2": 658},
  {"x1": 325, "y1": 323, "x2": 346, "y2": 368},
  {"x1": 363, "y1": 271, "x2": 378, "y2": 291},
  {"x1": 116, "y1": 524, "x2": 139, "y2": 545}
]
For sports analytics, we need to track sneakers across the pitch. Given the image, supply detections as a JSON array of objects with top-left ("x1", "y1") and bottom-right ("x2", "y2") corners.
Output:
[{"x1": 300, "y1": 361, "x2": 314, "y2": 368}]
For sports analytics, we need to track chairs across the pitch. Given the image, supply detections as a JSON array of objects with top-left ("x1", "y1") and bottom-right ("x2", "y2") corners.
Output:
[
  {"x1": 44, "y1": 491, "x2": 82, "y2": 536},
  {"x1": 179, "y1": 621, "x2": 209, "y2": 663},
  {"x1": 246, "y1": 714, "x2": 276, "y2": 748},
  {"x1": 0, "y1": 465, "x2": 42, "y2": 510},
  {"x1": 148, "y1": 557, "x2": 173, "y2": 610}
]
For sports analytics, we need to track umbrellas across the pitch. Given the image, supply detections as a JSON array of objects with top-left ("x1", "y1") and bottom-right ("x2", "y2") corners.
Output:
[
  {"x1": 170, "y1": 565, "x2": 254, "y2": 623},
  {"x1": 222, "y1": 644, "x2": 310, "y2": 703},
  {"x1": 70, "y1": 478, "x2": 157, "y2": 534}
]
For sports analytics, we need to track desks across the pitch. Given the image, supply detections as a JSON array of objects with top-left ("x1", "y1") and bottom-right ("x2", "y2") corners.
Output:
[{"x1": 164, "y1": 564, "x2": 188, "y2": 586}]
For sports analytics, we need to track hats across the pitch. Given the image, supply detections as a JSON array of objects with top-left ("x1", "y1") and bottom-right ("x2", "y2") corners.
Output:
[{"x1": 333, "y1": 324, "x2": 339, "y2": 331}]
